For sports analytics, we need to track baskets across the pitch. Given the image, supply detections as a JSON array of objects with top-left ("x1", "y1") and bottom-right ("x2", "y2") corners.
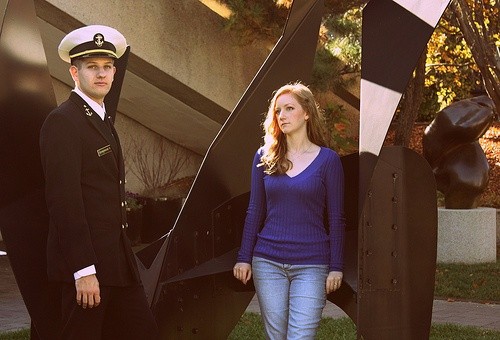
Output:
[
  {"x1": 122, "y1": 196, "x2": 153, "y2": 236},
  {"x1": 142, "y1": 175, "x2": 197, "y2": 229}
]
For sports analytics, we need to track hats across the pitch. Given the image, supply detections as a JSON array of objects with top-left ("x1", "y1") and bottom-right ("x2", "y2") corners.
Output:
[{"x1": 57, "y1": 25, "x2": 127, "y2": 64}]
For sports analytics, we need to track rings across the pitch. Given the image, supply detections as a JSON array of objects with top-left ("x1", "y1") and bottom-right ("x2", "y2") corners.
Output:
[{"x1": 334, "y1": 283, "x2": 337, "y2": 286}]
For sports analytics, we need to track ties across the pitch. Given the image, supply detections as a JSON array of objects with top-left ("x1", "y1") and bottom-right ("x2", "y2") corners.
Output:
[{"x1": 103, "y1": 112, "x2": 116, "y2": 142}]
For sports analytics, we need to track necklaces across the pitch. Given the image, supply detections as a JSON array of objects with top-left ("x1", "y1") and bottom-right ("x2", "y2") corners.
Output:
[{"x1": 291, "y1": 140, "x2": 313, "y2": 162}]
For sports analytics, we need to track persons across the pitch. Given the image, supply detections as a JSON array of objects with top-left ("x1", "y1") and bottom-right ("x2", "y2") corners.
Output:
[
  {"x1": 40, "y1": 25, "x2": 156, "y2": 340},
  {"x1": 233, "y1": 81, "x2": 345, "y2": 340}
]
